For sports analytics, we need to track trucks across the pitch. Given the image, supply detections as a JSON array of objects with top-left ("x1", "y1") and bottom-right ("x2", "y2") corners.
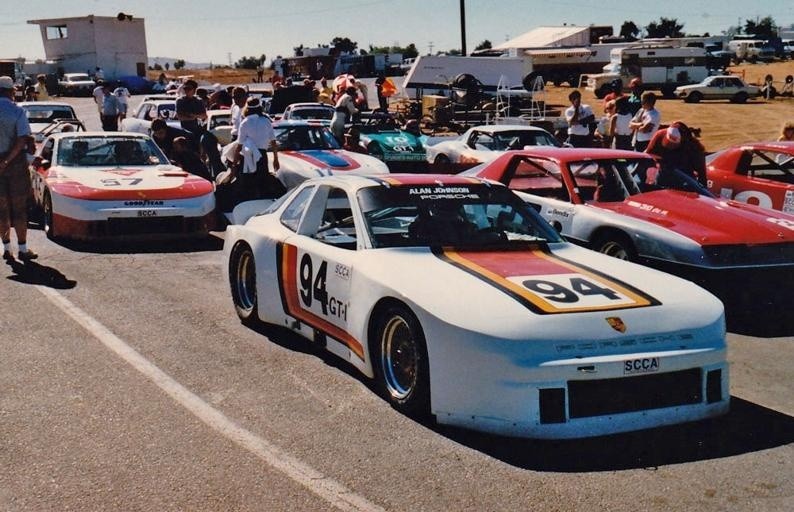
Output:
[{"x1": 584, "y1": 42, "x2": 710, "y2": 99}]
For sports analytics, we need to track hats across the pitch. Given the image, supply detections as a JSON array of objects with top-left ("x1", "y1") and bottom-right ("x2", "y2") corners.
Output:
[
  {"x1": 246, "y1": 102, "x2": 262, "y2": 108},
  {"x1": 629, "y1": 78, "x2": 640, "y2": 88},
  {"x1": 0, "y1": 76, "x2": 16, "y2": 88},
  {"x1": 662, "y1": 122, "x2": 691, "y2": 149},
  {"x1": 612, "y1": 79, "x2": 621, "y2": 86}
]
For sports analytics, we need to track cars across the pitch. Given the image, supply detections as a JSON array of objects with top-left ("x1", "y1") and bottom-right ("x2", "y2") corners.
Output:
[
  {"x1": 57, "y1": 72, "x2": 97, "y2": 96},
  {"x1": 672, "y1": 75, "x2": 762, "y2": 104}
]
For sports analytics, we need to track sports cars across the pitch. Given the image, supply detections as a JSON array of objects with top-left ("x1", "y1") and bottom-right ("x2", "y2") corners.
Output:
[
  {"x1": 453, "y1": 146, "x2": 793, "y2": 304},
  {"x1": 644, "y1": 140, "x2": 794, "y2": 220},
  {"x1": 26, "y1": 130, "x2": 218, "y2": 244},
  {"x1": 220, "y1": 171, "x2": 731, "y2": 441},
  {"x1": 18, "y1": 93, "x2": 597, "y2": 213}
]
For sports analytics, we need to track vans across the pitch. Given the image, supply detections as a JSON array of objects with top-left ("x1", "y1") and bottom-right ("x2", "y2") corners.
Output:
[{"x1": 729, "y1": 39, "x2": 777, "y2": 64}]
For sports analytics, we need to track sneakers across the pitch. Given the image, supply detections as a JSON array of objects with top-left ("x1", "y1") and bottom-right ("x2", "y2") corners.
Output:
[
  {"x1": 4, "y1": 251, "x2": 13, "y2": 258},
  {"x1": 19, "y1": 249, "x2": 37, "y2": 259}
]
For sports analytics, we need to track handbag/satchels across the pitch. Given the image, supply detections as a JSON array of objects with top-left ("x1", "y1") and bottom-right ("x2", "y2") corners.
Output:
[{"x1": 616, "y1": 135, "x2": 632, "y2": 150}]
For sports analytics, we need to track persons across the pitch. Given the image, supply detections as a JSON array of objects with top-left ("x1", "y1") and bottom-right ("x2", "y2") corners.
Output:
[
  {"x1": 257, "y1": 62, "x2": 293, "y2": 89},
  {"x1": 565, "y1": 77, "x2": 658, "y2": 151},
  {"x1": 776, "y1": 120, "x2": 794, "y2": 143},
  {"x1": 645, "y1": 121, "x2": 708, "y2": 190},
  {"x1": 0, "y1": 73, "x2": 40, "y2": 265},
  {"x1": 67, "y1": 141, "x2": 136, "y2": 166},
  {"x1": 15, "y1": 74, "x2": 49, "y2": 102},
  {"x1": 93, "y1": 69, "x2": 130, "y2": 132},
  {"x1": 151, "y1": 72, "x2": 279, "y2": 202},
  {"x1": 306, "y1": 72, "x2": 388, "y2": 144}
]
